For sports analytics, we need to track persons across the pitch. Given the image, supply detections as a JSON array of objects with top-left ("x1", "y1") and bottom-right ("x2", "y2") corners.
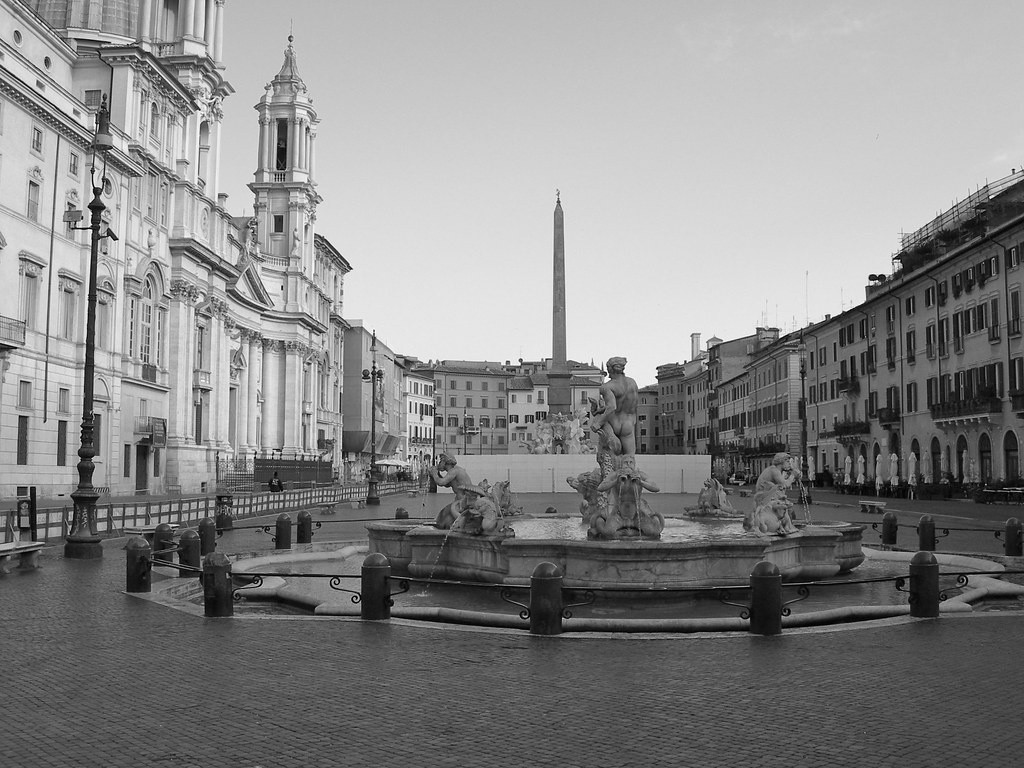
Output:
[
  {"x1": 596, "y1": 454, "x2": 661, "y2": 537},
  {"x1": 426, "y1": 454, "x2": 472, "y2": 529},
  {"x1": 590, "y1": 357, "x2": 639, "y2": 457},
  {"x1": 245, "y1": 228, "x2": 254, "y2": 254},
  {"x1": 396, "y1": 470, "x2": 408, "y2": 482},
  {"x1": 269, "y1": 472, "x2": 283, "y2": 492},
  {"x1": 754, "y1": 452, "x2": 801, "y2": 531},
  {"x1": 520, "y1": 438, "x2": 552, "y2": 454},
  {"x1": 579, "y1": 439, "x2": 596, "y2": 455},
  {"x1": 291, "y1": 228, "x2": 302, "y2": 253}
]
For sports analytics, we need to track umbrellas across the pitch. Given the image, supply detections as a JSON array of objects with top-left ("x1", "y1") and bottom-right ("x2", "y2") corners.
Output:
[
  {"x1": 375, "y1": 459, "x2": 410, "y2": 474},
  {"x1": 844, "y1": 454, "x2": 883, "y2": 496},
  {"x1": 940, "y1": 450, "x2": 949, "y2": 484},
  {"x1": 713, "y1": 457, "x2": 804, "y2": 480},
  {"x1": 922, "y1": 451, "x2": 934, "y2": 484},
  {"x1": 807, "y1": 456, "x2": 816, "y2": 487},
  {"x1": 962, "y1": 450, "x2": 971, "y2": 484},
  {"x1": 890, "y1": 453, "x2": 898, "y2": 484},
  {"x1": 908, "y1": 452, "x2": 918, "y2": 487}
]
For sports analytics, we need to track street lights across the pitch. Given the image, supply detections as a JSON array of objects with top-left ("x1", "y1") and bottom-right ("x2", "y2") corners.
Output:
[
  {"x1": 797, "y1": 328, "x2": 813, "y2": 504},
  {"x1": 362, "y1": 330, "x2": 384, "y2": 507},
  {"x1": 63, "y1": 92, "x2": 114, "y2": 561}
]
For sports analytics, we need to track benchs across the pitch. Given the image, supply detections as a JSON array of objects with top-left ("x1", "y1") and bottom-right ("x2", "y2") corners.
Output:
[
  {"x1": 406, "y1": 490, "x2": 419, "y2": 498},
  {"x1": 123, "y1": 524, "x2": 180, "y2": 545},
  {"x1": 724, "y1": 488, "x2": 734, "y2": 495},
  {"x1": 0, "y1": 541, "x2": 45, "y2": 574},
  {"x1": 859, "y1": 501, "x2": 887, "y2": 514},
  {"x1": 739, "y1": 489, "x2": 752, "y2": 497},
  {"x1": 349, "y1": 497, "x2": 367, "y2": 508},
  {"x1": 318, "y1": 502, "x2": 339, "y2": 515}
]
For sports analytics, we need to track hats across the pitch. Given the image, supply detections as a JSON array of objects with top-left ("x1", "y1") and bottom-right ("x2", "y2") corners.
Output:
[{"x1": 274, "y1": 471, "x2": 279, "y2": 476}]
